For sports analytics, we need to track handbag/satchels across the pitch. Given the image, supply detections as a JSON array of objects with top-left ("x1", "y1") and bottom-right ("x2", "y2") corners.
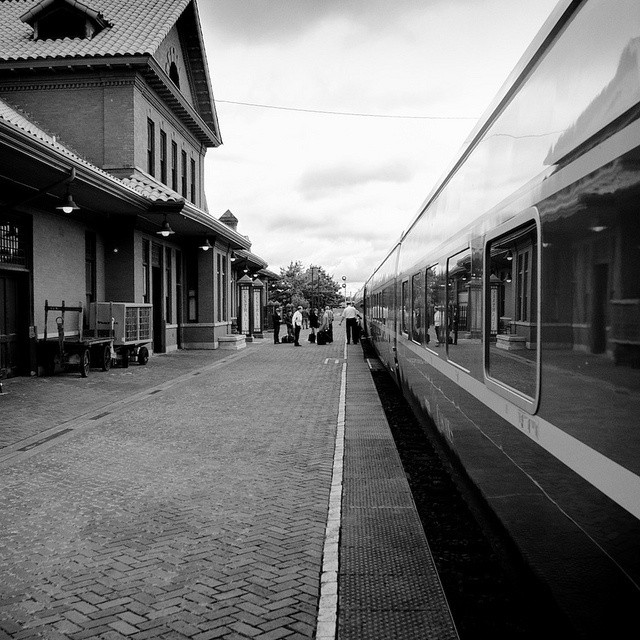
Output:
[{"x1": 308, "y1": 334, "x2": 315, "y2": 341}]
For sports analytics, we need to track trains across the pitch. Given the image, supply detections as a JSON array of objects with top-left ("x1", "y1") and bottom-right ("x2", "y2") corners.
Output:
[{"x1": 353, "y1": 0, "x2": 636, "y2": 637}]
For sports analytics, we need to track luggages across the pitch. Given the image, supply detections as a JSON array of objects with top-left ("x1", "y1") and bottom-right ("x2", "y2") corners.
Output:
[{"x1": 317, "y1": 331, "x2": 326, "y2": 345}]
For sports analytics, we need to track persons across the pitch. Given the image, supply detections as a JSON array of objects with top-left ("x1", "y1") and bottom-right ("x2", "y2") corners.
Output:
[
  {"x1": 303, "y1": 308, "x2": 309, "y2": 330},
  {"x1": 402, "y1": 304, "x2": 445, "y2": 348},
  {"x1": 311, "y1": 305, "x2": 335, "y2": 344},
  {"x1": 286, "y1": 310, "x2": 293, "y2": 337},
  {"x1": 293, "y1": 305, "x2": 303, "y2": 346},
  {"x1": 343, "y1": 301, "x2": 363, "y2": 345},
  {"x1": 272, "y1": 307, "x2": 282, "y2": 344}
]
